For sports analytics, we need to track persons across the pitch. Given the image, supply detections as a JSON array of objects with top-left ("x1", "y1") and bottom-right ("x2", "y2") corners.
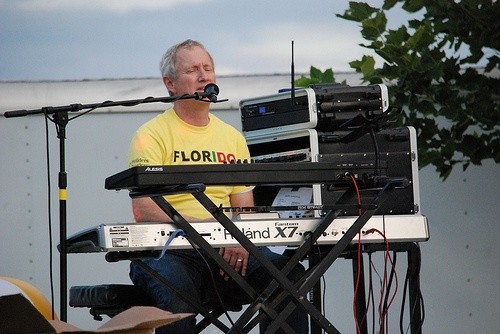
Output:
[{"x1": 129, "y1": 38, "x2": 309, "y2": 334}]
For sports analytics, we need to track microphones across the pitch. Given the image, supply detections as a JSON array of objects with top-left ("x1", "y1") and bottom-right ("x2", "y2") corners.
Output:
[{"x1": 204, "y1": 83, "x2": 219, "y2": 103}]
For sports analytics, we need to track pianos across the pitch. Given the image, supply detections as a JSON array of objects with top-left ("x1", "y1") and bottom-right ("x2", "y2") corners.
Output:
[
  {"x1": 56, "y1": 214, "x2": 432, "y2": 255},
  {"x1": 104, "y1": 161, "x2": 383, "y2": 191}
]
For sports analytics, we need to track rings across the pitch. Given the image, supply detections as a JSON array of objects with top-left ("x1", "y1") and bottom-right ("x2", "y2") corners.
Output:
[{"x1": 237, "y1": 258, "x2": 243, "y2": 262}]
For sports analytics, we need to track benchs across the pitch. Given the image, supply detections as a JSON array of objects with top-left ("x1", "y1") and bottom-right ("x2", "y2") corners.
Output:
[{"x1": 70, "y1": 284, "x2": 253, "y2": 327}]
